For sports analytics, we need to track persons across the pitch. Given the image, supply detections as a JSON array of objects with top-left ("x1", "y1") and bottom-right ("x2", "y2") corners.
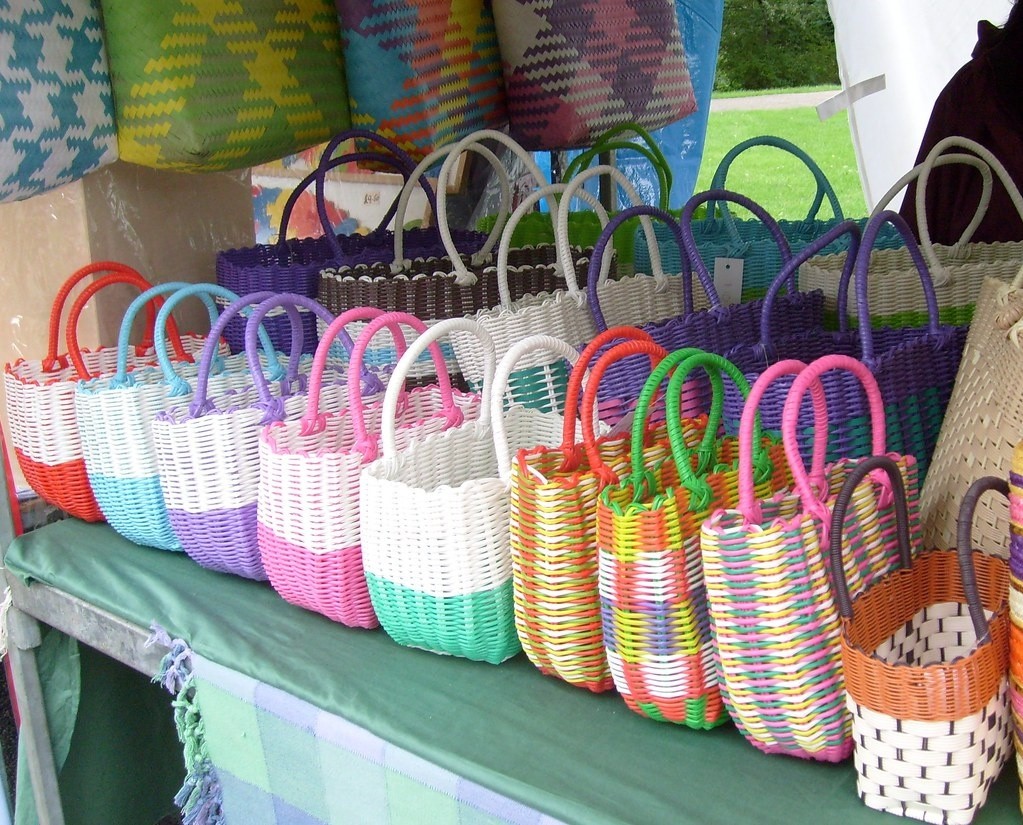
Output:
[{"x1": 900, "y1": 0, "x2": 1022, "y2": 247}]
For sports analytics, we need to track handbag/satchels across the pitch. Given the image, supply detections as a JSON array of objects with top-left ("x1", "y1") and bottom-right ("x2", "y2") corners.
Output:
[
  {"x1": 0, "y1": 0, "x2": 121, "y2": 197},
  {"x1": 102, "y1": 0, "x2": 350, "y2": 178},
  {"x1": 336, "y1": 0, "x2": 510, "y2": 173},
  {"x1": 3, "y1": 121, "x2": 1023, "y2": 825},
  {"x1": 495, "y1": 0, "x2": 697, "y2": 152}
]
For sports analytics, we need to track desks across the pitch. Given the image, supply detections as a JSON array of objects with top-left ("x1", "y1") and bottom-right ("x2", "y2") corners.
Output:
[{"x1": 1, "y1": 510, "x2": 1023, "y2": 825}]
